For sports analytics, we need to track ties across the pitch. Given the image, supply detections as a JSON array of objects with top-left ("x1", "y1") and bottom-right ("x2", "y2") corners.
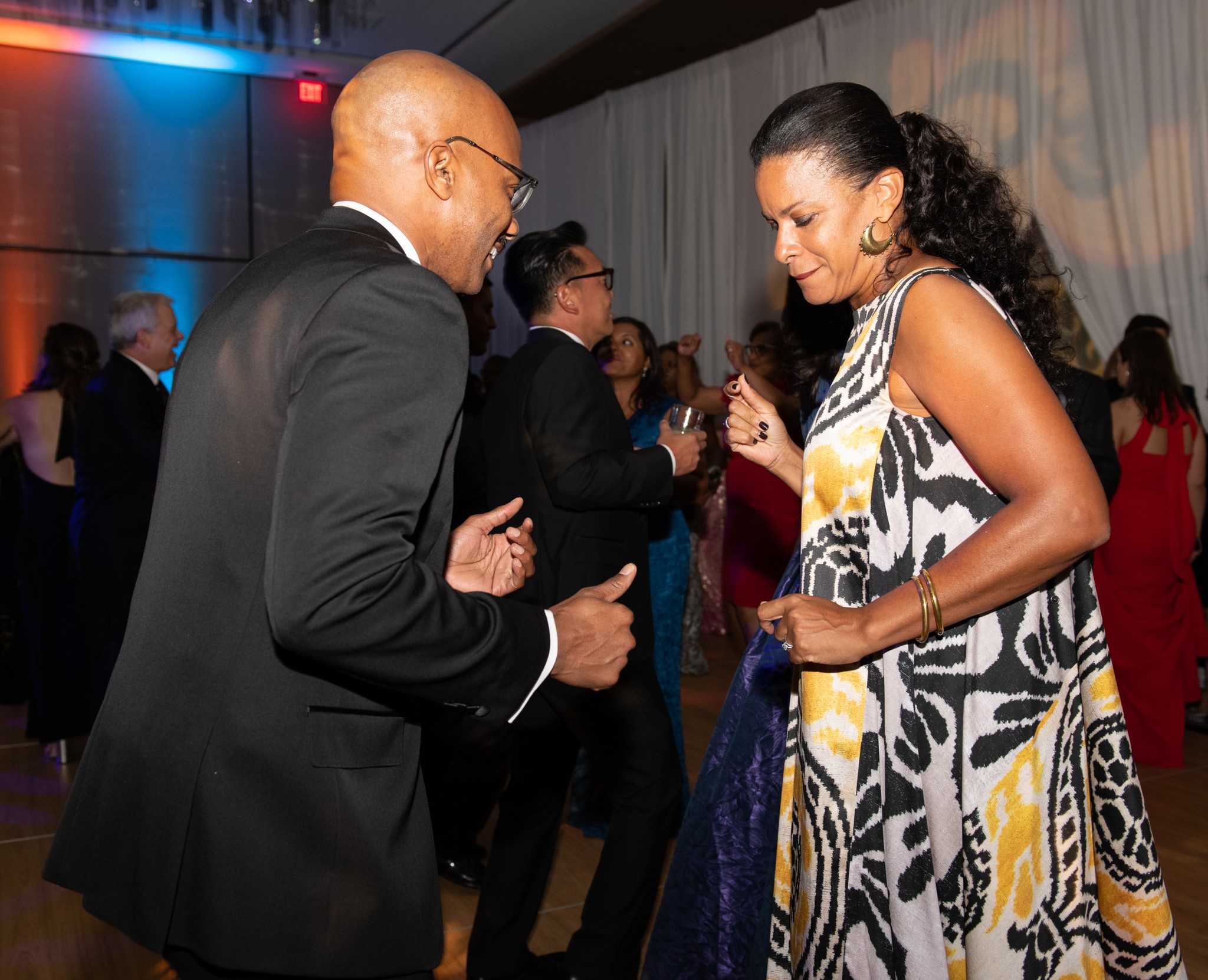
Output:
[{"x1": 155, "y1": 380, "x2": 169, "y2": 401}]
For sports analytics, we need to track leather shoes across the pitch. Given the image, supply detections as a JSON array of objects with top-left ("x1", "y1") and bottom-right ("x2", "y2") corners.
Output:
[{"x1": 436, "y1": 840, "x2": 491, "y2": 892}]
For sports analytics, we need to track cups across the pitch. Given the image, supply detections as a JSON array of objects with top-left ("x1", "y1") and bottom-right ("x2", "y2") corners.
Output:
[{"x1": 670, "y1": 404, "x2": 705, "y2": 434}]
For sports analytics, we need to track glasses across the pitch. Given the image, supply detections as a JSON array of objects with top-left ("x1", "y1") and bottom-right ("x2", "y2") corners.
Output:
[
  {"x1": 440, "y1": 136, "x2": 537, "y2": 214},
  {"x1": 554, "y1": 266, "x2": 615, "y2": 299},
  {"x1": 743, "y1": 339, "x2": 779, "y2": 359}
]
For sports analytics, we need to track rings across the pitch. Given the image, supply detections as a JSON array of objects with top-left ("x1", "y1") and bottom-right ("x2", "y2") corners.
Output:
[
  {"x1": 782, "y1": 635, "x2": 792, "y2": 651},
  {"x1": 723, "y1": 417, "x2": 730, "y2": 429}
]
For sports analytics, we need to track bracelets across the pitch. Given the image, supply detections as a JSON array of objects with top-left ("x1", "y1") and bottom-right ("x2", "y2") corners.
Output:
[
  {"x1": 920, "y1": 569, "x2": 944, "y2": 636},
  {"x1": 910, "y1": 576, "x2": 930, "y2": 643}
]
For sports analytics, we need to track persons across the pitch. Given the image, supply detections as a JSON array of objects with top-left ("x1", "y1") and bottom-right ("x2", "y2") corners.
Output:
[
  {"x1": 0, "y1": 224, "x2": 1208, "y2": 980},
  {"x1": 42, "y1": 49, "x2": 637, "y2": 980},
  {"x1": 723, "y1": 83, "x2": 1189, "y2": 980}
]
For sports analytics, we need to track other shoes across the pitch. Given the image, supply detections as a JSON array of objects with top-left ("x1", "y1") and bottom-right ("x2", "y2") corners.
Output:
[
  {"x1": 517, "y1": 946, "x2": 574, "y2": 980},
  {"x1": 42, "y1": 737, "x2": 71, "y2": 765}
]
